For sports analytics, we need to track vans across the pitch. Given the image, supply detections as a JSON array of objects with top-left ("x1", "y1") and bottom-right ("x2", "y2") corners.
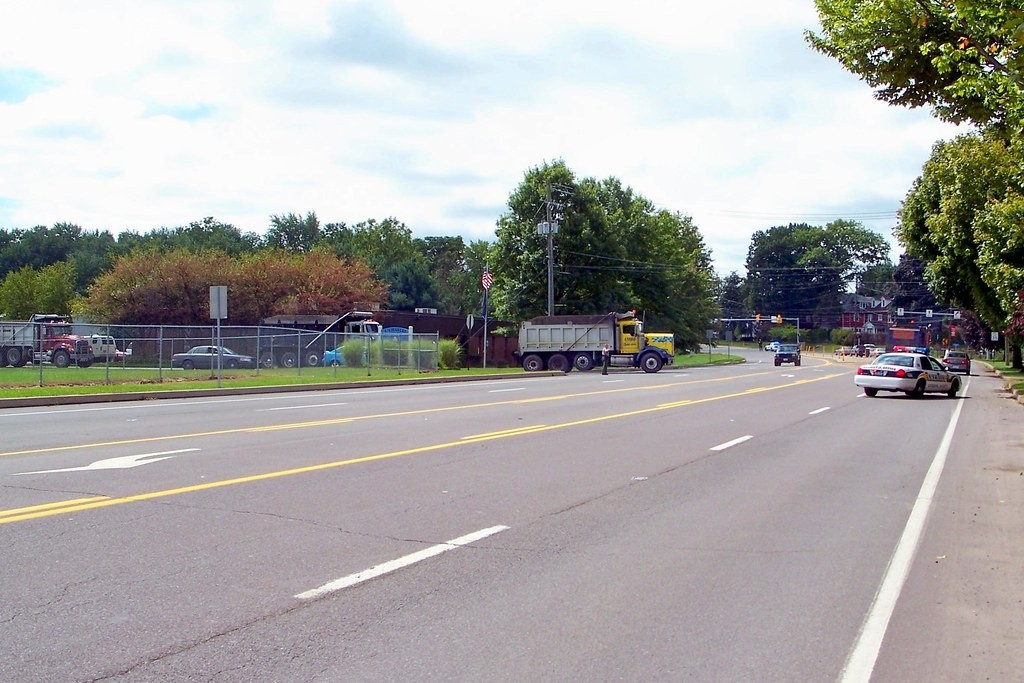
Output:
[
  {"x1": 83, "y1": 335, "x2": 117, "y2": 362},
  {"x1": 862, "y1": 343, "x2": 876, "y2": 356}
]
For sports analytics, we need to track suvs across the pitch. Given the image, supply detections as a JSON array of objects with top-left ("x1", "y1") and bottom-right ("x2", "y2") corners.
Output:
[
  {"x1": 850, "y1": 345, "x2": 867, "y2": 357},
  {"x1": 775, "y1": 343, "x2": 801, "y2": 367}
]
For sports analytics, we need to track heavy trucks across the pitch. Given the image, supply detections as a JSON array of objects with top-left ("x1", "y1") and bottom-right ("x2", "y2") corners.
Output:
[
  {"x1": 516, "y1": 310, "x2": 674, "y2": 376},
  {"x1": 255, "y1": 312, "x2": 381, "y2": 370},
  {"x1": 0, "y1": 313, "x2": 92, "y2": 367}
]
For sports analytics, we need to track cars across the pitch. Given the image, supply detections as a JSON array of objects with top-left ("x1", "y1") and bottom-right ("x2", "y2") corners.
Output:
[
  {"x1": 321, "y1": 343, "x2": 367, "y2": 369},
  {"x1": 172, "y1": 345, "x2": 256, "y2": 370},
  {"x1": 765, "y1": 341, "x2": 781, "y2": 352},
  {"x1": 853, "y1": 346, "x2": 963, "y2": 401},
  {"x1": 941, "y1": 351, "x2": 971, "y2": 375},
  {"x1": 870, "y1": 348, "x2": 887, "y2": 357},
  {"x1": 116, "y1": 349, "x2": 127, "y2": 362},
  {"x1": 835, "y1": 346, "x2": 852, "y2": 356}
]
